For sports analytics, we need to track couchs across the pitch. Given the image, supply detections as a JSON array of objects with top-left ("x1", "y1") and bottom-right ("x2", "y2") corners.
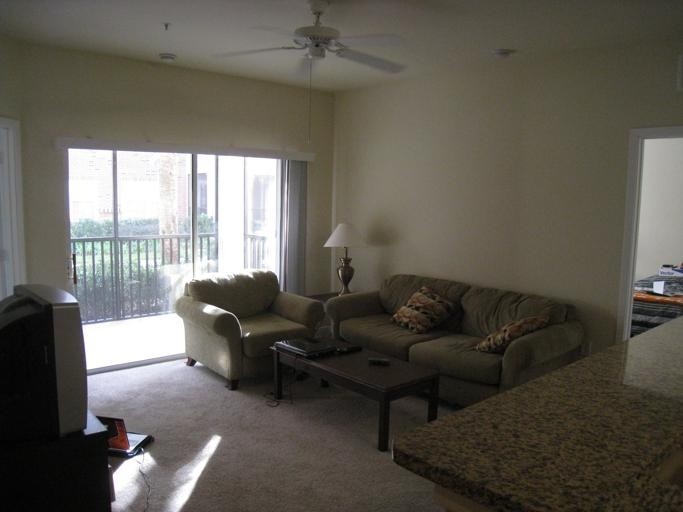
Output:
[{"x1": 326, "y1": 273, "x2": 587, "y2": 408}]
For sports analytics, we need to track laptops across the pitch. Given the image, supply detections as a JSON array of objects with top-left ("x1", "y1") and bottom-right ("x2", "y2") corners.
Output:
[
  {"x1": 96, "y1": 416, "x2": 155, "y2": 457},
  {"x1": 274, "y1": 336, "x2": 336, "y2": 359}
]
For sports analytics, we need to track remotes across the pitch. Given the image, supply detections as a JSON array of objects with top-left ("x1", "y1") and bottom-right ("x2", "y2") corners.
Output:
[{"x1": 368, "y1": 357, "x2": 391, "y2": 365}]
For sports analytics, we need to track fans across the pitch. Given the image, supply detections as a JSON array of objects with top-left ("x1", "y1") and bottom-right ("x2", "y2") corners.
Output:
[{"x1": 216, "y1": 2, "x2": 417, "y2": 78}]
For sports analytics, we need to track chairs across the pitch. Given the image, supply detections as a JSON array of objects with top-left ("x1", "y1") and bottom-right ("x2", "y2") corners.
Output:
[{"x1": 174, "y1": 269, "x2": 323, "y2": 394}]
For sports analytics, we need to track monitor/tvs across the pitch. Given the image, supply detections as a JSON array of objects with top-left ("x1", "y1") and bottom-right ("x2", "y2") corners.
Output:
[{"x1": 0, "y1": 284, "x2": 87, "y2": 441}]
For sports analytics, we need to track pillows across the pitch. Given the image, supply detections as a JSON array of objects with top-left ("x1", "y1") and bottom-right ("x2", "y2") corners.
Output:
[
  {"x1": 392, "y1": 287, "x2": 455, "y2": 334},
  {"x1": 474, "y1": 314, "x2": 550, "y2": 353}
]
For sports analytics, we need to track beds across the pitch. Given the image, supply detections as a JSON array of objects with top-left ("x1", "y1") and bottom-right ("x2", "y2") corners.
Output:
[{"x1": 632, "y1": 265, "x2": 683, "y2": 334}]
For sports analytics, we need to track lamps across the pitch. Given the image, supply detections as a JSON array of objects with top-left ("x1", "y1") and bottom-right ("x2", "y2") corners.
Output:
[{"x1": 322, "y1": 221, "x2": 364, "y2": 294}]
